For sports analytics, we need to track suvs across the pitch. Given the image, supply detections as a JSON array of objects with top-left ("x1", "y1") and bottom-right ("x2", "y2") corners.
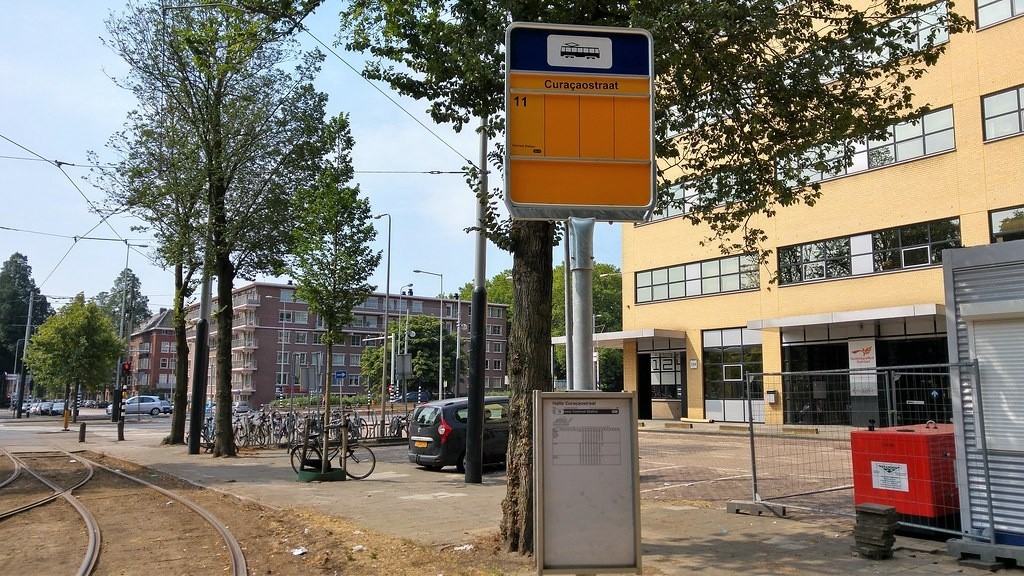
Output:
[{"x1": 407, "y1": 395, "x2": 510, "y2": 474}]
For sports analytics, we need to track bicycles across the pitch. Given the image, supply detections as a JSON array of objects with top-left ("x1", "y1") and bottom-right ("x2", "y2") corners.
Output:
[
  {"x1": 290, "y1": 420, "x2": 376, "y2": 480},
  {"x1": 185, "y1": 403, "x2": 412, "y2": 453}
]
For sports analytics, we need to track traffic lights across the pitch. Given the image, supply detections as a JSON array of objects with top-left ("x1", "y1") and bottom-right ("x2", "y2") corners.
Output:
[{"x1": 121, "y1": 363, "x2": 131, "y2": 377}]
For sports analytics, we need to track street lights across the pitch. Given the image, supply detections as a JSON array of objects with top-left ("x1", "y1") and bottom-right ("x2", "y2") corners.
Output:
[
  {"x1": 11, "y1": 339, "x2": 33, "y2": 405},
  {"x1": 412, "y1": 269, "x2": 443, "y2": 400},
  {"x1": 399, "y1": 283, "x2": 414, "y2": 402},
  {"x1": 373, "y1": 212, "x2": 392, "y2": 437},
  {"x1": 452, "y1": 291, "x2": 461, "y2": 398}
]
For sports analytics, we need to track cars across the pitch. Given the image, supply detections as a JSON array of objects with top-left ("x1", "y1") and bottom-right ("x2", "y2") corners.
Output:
[
  {"x1": 107, "y1": 395, "x2": 171, "y2": 416},
  {"x1": 276, "y1": 388, "x2": 284, "y2": 393},
  {"x1": 19, "y1": 398, "x2": 108, "y2": 416},
  {"x1": 231, "y1": 401, "x2": 251, "y2": 416}
]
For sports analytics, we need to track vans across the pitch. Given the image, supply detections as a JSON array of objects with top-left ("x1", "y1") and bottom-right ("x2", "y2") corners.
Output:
[{"x1": 393, "y1": 392, "x2": 429, "y2": 403}]
